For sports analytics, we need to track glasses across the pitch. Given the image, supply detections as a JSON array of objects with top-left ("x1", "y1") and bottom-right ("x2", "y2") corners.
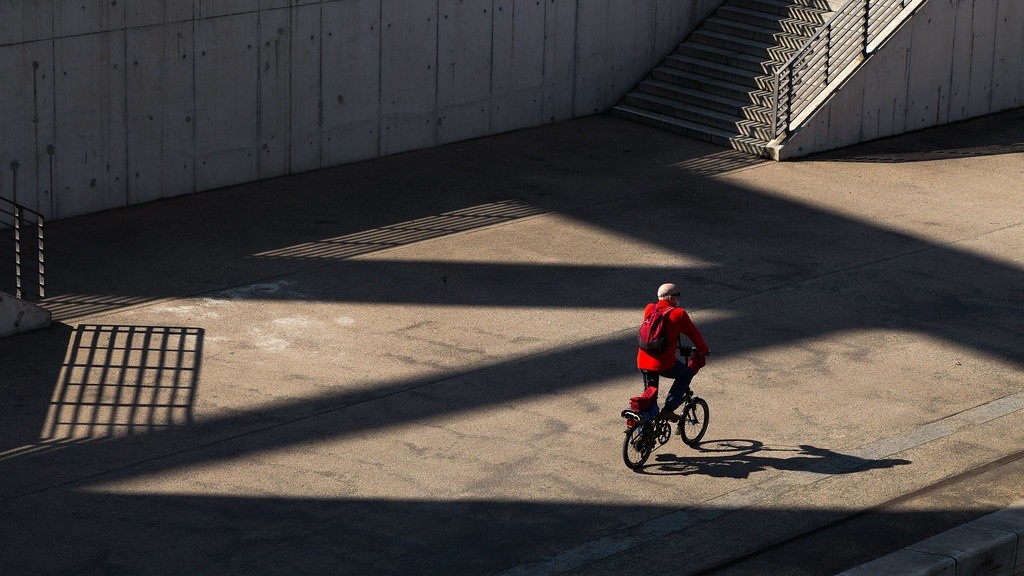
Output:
[{"x1": 670, "y1": 292, "x2": 680, "y2": 297}]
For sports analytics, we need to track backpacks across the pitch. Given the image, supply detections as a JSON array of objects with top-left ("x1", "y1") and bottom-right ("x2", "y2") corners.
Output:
[{"x1": 639, "y1": 304, "x2": 675, "y2": 357}]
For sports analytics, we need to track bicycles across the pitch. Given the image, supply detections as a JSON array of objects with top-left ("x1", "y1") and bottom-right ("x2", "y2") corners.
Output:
[{"x1": 621, "y1": 345, "x2": 712, "y2": 469}]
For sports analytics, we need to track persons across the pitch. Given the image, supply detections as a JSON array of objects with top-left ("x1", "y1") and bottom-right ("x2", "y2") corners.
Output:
[{"x1": 637, "y1": 283, "x2": 709, "y2": 436}]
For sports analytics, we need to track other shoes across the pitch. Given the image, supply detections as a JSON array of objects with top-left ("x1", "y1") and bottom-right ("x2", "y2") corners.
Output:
[{"x1": 661, "y1": 408, "x2": 680, "y2": 421}]
